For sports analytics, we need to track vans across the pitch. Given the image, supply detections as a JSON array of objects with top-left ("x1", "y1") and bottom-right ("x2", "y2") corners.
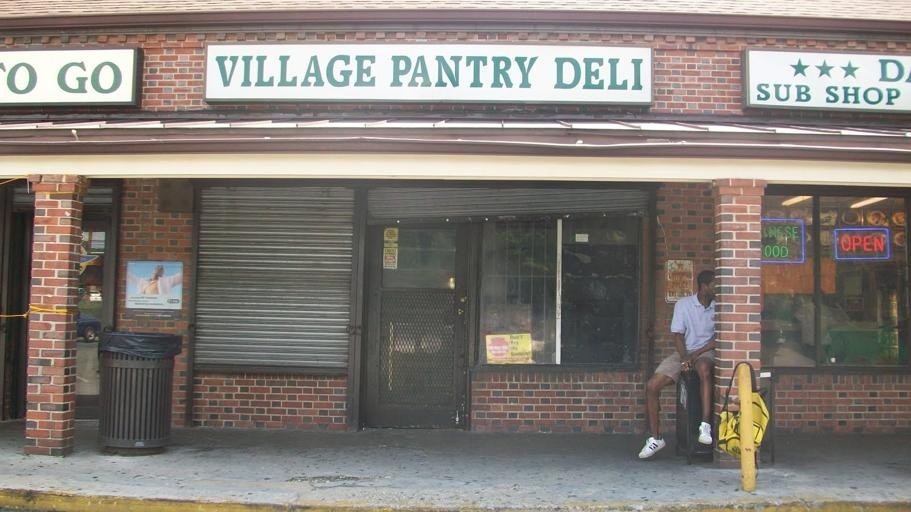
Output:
[{"x1": 86, "y1": 289, "x2": 102, "y2": 304}]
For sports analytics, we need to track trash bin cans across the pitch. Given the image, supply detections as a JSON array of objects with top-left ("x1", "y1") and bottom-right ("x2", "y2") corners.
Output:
[
  {"x1": 98, "y1": 331, "x2": 183, "y2": 456},
  {"x1": 827, "y1": 322, "x2": 892, "y2": 364}
]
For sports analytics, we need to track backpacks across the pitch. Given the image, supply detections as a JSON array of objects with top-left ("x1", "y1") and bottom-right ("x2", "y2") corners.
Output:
[{"x1": 716, "y1": 388, "x2": 770, "y2": 459}]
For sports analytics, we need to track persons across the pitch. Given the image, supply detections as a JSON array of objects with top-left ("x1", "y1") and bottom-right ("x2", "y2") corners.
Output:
[
  {"x1": 125, "y1": 264, "x2": 182, "y2": 298},
  {"x1": 792, "y1": 289, "x2": 850, "y2": 364},
  {"x1": 637, "y1": 269, "x2": 717, "y2": 460}
]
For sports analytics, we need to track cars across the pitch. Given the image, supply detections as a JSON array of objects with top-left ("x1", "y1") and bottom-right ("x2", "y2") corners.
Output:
[
  {"x1": 75, "y1": 305, "x2": 101, "y2": 342},
  {"x1": 78, "y1": 288, "x2": 85, "y2": 299}
]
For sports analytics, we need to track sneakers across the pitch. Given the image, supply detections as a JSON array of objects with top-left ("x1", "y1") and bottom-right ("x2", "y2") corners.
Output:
[
  {"x1": 698, "y1": 421, "x2": 713, "y2": 445},
  {"x1": 638, "y1": 434, "x2": 666, "y2": 459}
]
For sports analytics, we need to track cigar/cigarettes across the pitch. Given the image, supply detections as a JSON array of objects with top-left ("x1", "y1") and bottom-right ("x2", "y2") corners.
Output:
[{"x1": 682, "y1": 361, "x2": 689, "y2": 365}]
